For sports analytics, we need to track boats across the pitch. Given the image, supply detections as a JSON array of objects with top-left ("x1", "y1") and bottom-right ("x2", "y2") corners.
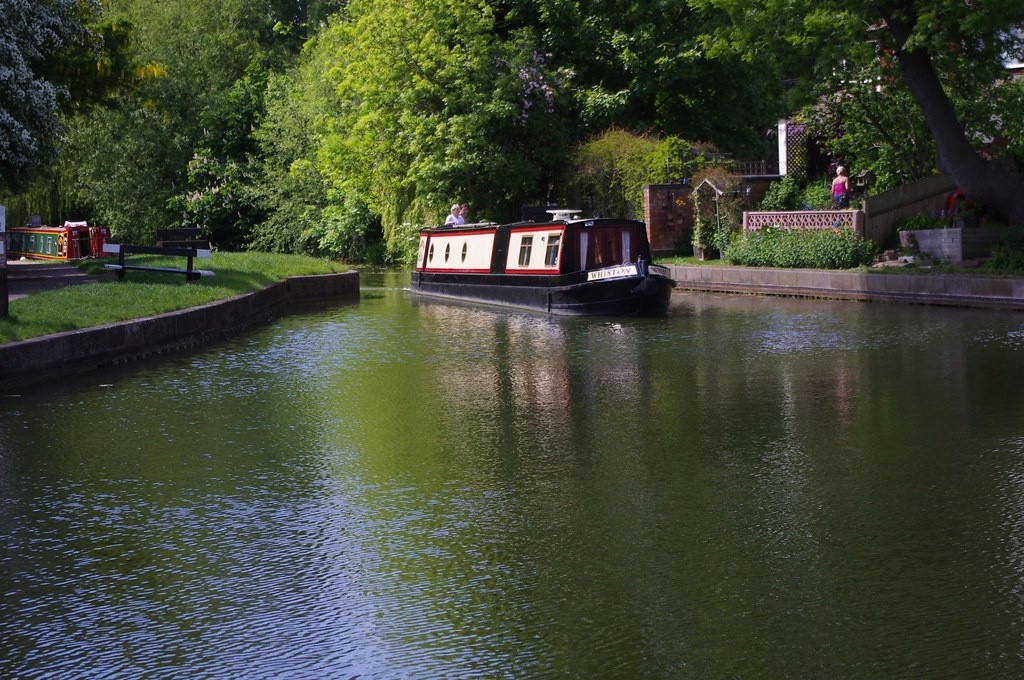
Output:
[{"x1": 409, "y1": 210, "x2": 678, "y2": 319}]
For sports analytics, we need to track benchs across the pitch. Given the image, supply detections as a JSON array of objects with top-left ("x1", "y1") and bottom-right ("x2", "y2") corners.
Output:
[
  {"x1": 101, "y1": 244, "x2": 215, "y2": 282},
  {"x1": 157, "y1": 229, "x2": 207, "y2": 250}
]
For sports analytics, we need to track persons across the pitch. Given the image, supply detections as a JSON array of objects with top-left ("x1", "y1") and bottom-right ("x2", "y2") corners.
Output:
[
  {"x1": 445, "y1": 204, "x2": 464, "y2": 226},
  {"x1": 832, "y1": 166, "x2": 850, "y2": 207},
  {"x1": 459, "y1": 203, "x2": 469, "y2": 224}
]
